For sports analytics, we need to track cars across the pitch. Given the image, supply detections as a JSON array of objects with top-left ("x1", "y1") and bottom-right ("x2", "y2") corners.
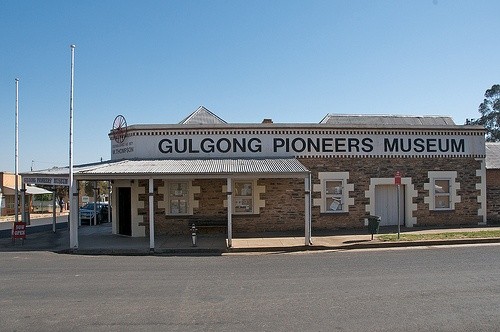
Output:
[{"x1": 80, "y1": 201, "x2": 112, "y2": 222}]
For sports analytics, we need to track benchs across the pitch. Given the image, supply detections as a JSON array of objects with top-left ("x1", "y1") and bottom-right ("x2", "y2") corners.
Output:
[{"x1": 187, "y1": 215, "x2": 233, "y2": 235}]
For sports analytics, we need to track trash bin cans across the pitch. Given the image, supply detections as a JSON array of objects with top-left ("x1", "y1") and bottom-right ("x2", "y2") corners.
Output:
[{"x1": 368, "y1": 216, "x2": 381, "y2": 234}]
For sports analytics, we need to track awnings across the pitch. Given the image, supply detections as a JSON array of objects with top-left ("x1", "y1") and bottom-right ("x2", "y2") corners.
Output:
[{"x1": 17, "y1": 158, "x2": 313, "y2": 254}]
